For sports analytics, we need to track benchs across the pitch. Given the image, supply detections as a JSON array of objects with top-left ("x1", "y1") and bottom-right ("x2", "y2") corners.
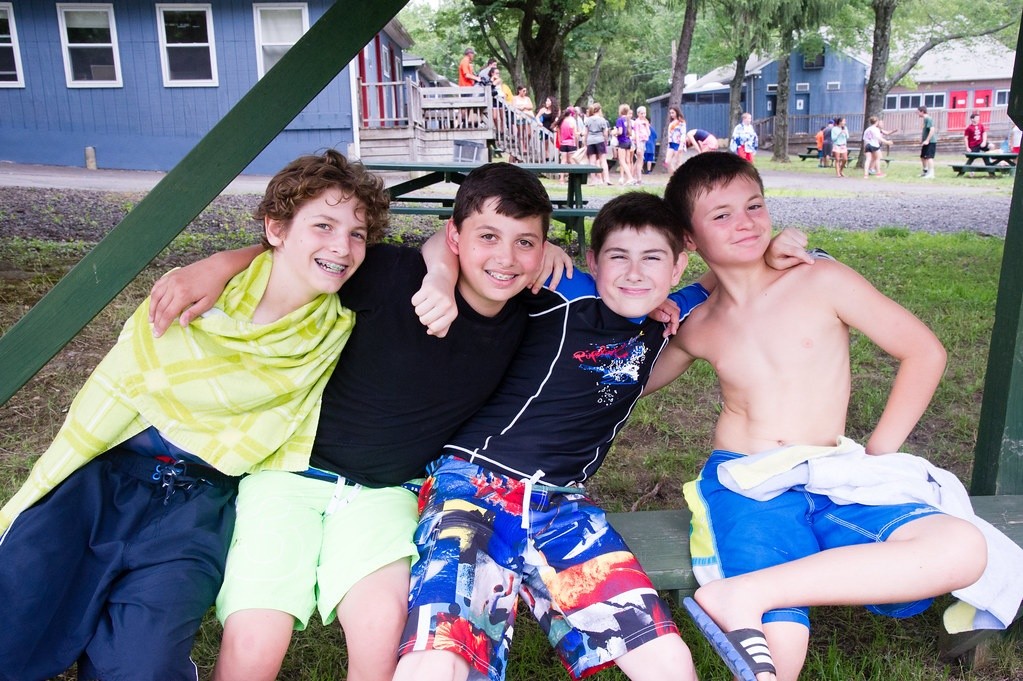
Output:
[
  {"x1": 797, "y1": 154, "x2": 821, "y2": 158},
  {"x1": 371, "y1": 196, "x2": 601, "y2": 220},
  {"x1": 610, "y1": 492, "x2": 1023, "y2": 622},
  {"x1": 851, "y1": 156, "x2": 894, "y2": 163},
  {"x1": 948, "y1": 164, "x2": 1016, "y2": 173}
]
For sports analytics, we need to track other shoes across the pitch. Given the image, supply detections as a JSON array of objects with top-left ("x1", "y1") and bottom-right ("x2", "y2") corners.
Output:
[
  {"x1": 876, "y1": 171, "x2": 887, "y2": 177},
  {"x1": 865, "y1": 173, "x2": 867, "y2": 179}
]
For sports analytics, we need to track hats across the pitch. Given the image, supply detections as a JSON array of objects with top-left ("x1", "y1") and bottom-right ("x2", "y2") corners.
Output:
[
  {"x1": 465, "y1": 48, "x2": 474, "y2": 55},
  {"x1": 567, "y1": 107, "x2": 576, "y2": 114}
]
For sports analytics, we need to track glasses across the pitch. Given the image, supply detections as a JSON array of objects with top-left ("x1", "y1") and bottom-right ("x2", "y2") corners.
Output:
[{"x1": 521, "y1": 90, "x2": 528, "y2": 92}]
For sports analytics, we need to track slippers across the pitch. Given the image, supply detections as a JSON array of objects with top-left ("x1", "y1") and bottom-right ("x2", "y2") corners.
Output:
[{"x1": 683, "y1": 597, "x2": 776, "y2": 681}]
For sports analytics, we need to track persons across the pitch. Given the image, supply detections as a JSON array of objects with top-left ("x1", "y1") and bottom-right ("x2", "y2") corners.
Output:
[
  {"x1": 862, "y1": 117, "x2": 897, "y2": 179},
  {"x1": 665, "y1": 106, "x2": 686, "y2": 174},
  {"x1": 965, "y1": 113, "x2": 990, "y2": 153},
  {"x1": 459, "y1": 48, "x2": 559, "y2": 163},
  {"x1": 729, "y1": 113, "x2": 758, "y2": 162},
  {"x1": 147, "y1": 161, "x2": 555, "y2": 681},
  {"x1": 552, "y1": 103, "x2": 613, "y2": 185},
  {"x1": 918, "y1": 107, "x2": 937, "y2": 179},
  {"x1": 614, "y1": 103, "x2": 657, "y2": 186},
  {"x1": 1009, "y1": 125, "x2": 1023, "y2": 154},
  {"x1": 0, "y1": 150, "x2": 573, "y2": 681},
  {"x1": 394, "y1": 193, "x2": 815, "y2": 681},
  {"x1": 637, "y1": 151, "x2": 989, "y2": 681},
  {"x1": 816, "y1": 120, "x2": 834, "y2": 168},
  {"x1": 685, "y1": 129, "x2": 719, "y2": 154},
  {"x1": 831, "y1": 117, "x2": 850, "y2": 178}
]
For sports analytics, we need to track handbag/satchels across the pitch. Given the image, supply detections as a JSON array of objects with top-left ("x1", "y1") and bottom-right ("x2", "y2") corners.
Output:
[{"x1": 572, "y1": 147, "x2": 589, "y2": 165}]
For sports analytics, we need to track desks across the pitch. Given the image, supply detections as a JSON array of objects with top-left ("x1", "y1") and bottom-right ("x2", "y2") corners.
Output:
[
  {"x1": 957, "y1": 153, "x2": 1019, "y2": 177},
  {"x1": 803, "y1": 147, "x2": 861, "y2": 162},
  {"x1": 358, "y1": 161, "x2": 604, "y2": 259}
]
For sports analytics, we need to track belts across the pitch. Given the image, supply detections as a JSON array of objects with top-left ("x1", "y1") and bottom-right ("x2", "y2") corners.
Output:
[
  {"x1": 290, "y1": 467, "x2": 421, "y2": 494},
  {"x1": 100, "y1": 447, "x2": 240, "y2": 505}
]
